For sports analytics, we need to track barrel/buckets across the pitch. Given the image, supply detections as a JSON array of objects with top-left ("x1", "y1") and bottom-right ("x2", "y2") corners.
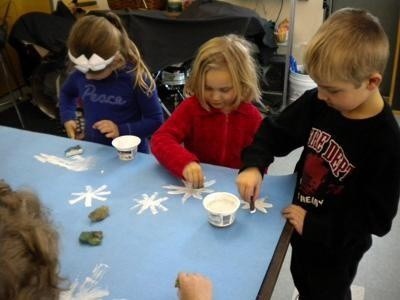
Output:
[{"x1": 288, "y1": 64, "x2": 318, "y2": 105}]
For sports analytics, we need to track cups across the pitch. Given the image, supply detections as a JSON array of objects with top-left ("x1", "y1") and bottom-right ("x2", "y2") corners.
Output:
[
  {"x1": 112, "y1": 135, "x2": 141, "y2": 161},
  {"x1": 203, "y1": 191, "x2": 240, "y2": 227}
]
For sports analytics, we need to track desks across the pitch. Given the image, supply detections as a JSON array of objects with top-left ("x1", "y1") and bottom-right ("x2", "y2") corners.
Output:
[{"x1": 0, "y1": 125, "x2": 299, "y2": 300}]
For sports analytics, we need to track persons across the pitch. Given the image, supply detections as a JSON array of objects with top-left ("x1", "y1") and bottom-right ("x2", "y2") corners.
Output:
[
  {"x1": 0, "y1": 177, "x2": 214, "y2": 300},
  {"x1": 235, "y1": 8, "x2": 400, "y2": 299},
  {"x1": 58, "y1": 10, "x2": 165, "y2": 156},
  {"x1": 149, "y1": 34, "x2": 267, "y2": 187}
]
